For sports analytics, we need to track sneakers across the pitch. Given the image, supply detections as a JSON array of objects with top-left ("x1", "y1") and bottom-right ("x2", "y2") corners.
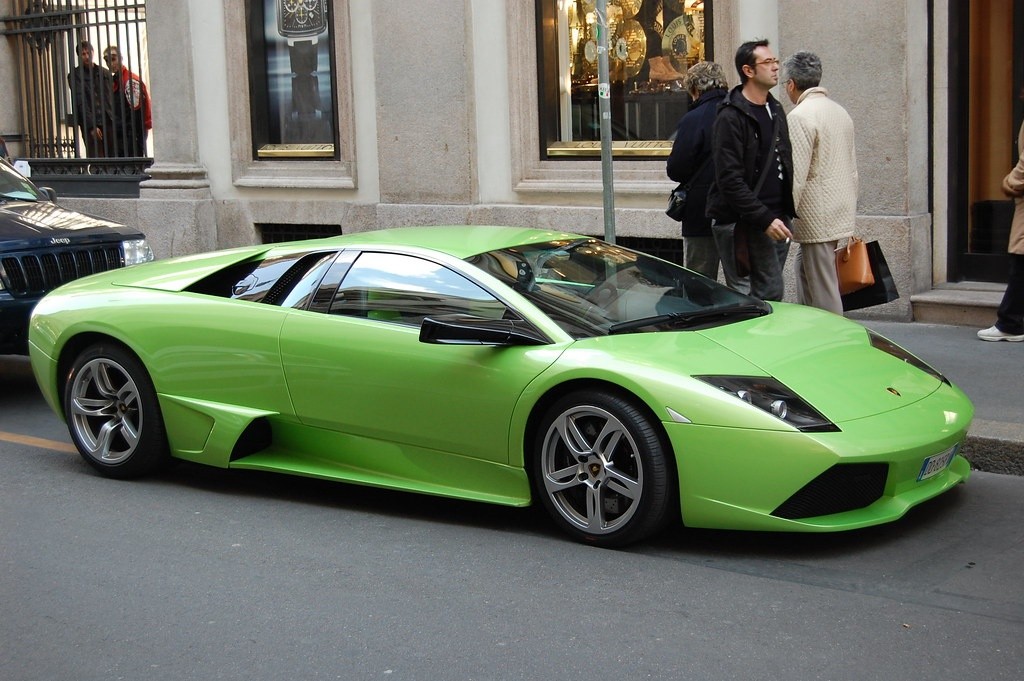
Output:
[{"x1": 976, "y1": 324, "x2": 1024, "y2": 341}]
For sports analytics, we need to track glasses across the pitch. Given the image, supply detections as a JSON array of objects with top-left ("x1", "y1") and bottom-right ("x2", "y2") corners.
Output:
[{"x1": 749, "y1": 58, "x2": 780, "y2": 66}]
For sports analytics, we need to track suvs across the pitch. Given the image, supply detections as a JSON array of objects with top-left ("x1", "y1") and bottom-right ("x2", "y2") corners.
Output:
[{"x1": 0, "y1": 153, "x2": 157, "y2": 356}]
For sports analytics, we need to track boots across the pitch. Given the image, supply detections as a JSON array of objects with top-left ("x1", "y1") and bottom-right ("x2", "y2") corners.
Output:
[{"x1": 648, "y1": 55, "x2": 685, "y2": 80}]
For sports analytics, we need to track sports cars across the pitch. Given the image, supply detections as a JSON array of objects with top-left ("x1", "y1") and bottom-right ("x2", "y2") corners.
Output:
[{"x1": 26, "y1": 224, "x2": 975, "y2": 552}]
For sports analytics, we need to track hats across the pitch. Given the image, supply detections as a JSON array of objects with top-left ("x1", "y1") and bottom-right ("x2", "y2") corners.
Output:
[{"x1": 103, "y1": 45, "x2": 118, "y2": 58}]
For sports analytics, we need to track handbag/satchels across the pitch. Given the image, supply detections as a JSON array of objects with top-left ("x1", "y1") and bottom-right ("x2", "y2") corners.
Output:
[
  {"x1": 664, "y1": 179, "x2": 696, "y2": 222},
  {"x1": 834, "y1": 234, "x2": 900, "y2": 311}
]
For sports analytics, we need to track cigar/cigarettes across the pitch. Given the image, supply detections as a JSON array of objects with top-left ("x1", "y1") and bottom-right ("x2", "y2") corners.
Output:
[{"x1": 786, "y1": 237, "x2": 789, "y2": 243}]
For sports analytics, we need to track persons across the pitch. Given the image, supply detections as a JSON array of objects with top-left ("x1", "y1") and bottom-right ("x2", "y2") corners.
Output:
[
  {"x1": 67, "y1": 40, "x2": 151, "y2": 175},
  {"x1": 666, "y1": 37, "x2": 859, "y2": 317},
  {"x1": 976, "y1": 121, "x2": 1024, "y2": 341}
]
